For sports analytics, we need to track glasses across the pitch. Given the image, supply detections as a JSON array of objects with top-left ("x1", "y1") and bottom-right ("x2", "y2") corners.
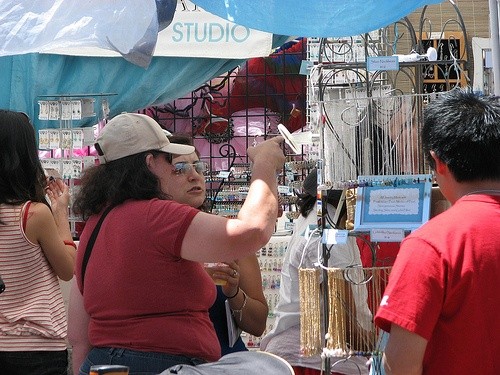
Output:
[{"x1": 168, "y1": 162, "x2": 204, "y2": 175}]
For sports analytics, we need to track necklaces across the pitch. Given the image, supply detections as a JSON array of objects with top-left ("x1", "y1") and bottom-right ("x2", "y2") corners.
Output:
[
  {"x1": 299, "y1": 267, "x2": 322, "y2": 357},
  {"x1": 320, "y1": 267, "x2": 354, "y2": 358},
  {"x1": 357, "y1": 97, "x2": 381, "y2": 187},
  {"x1": 316, "y1": 100, "x2": 339, "y2": 191},
  {"x1": 396, "y1": 95, "x2": 420, "y2": 186},
  {"x1": 336, "y1": 98, "x2": 359, "y2": 189},
  {"x1": 378, "y1": 96, "x2": 398, "y2": 189},
  {"x1": 418, "y1": 92, "x2": 437, "y2": 184},
  {"x1": 351, "y1": 266, "x2": 393, "y2": 357}
]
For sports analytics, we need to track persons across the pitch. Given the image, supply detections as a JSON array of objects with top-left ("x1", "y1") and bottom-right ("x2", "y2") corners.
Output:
[
  {"x1": 74, "y1": 111, "x2": 287, "y2": 375},
  {"x1": 259, "y1": 165, "x2": 372, "y2": 375},
  {"x1": 161, "y1": 135, "x2": 270, "y2": 356},
  {"x1": 0, "y1": 110, "x2": 77, "y2": 375},
  {"x1": 375, "y1": 90, "x2": 500, "y2": 375}
]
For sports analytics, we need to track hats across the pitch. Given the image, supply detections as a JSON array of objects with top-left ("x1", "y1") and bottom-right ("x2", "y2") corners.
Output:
[
  {"x1": 93, "y1": 112, "x2": 195, "y2": 165},
  {"x1": 304, "y1": 171, "x2": 348, "y2": 211}
]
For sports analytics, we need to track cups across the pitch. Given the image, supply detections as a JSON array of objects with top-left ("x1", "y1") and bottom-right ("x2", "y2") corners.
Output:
[{"x1": 203, "y1": 263, "x2": 229, "y2": 286}]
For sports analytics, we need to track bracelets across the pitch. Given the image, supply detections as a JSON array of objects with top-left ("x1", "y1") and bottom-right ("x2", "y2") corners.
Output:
[
  {"x1": 231, "y1": 289, "x2": 246, "y2": 312},
  {"x1": 229, "y1": 286, "x2": 239, "y2": 298},
  {"x1": 62, "y1": 240, "x2": 77, "y2": 250}
]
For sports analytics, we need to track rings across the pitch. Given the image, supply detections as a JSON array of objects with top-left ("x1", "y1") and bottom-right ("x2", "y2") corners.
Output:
[
  {"x1": 232, "y1": 270, "x2": 237, "y2": 277},
  {"x1": 58, "y1": 193, "x2": 62, "y2": 197}
]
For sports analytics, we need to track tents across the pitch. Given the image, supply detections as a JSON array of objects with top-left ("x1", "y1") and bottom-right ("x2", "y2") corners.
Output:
[{"x1": 0, "y1": 0, "x2": 445, "y2": 130}]
